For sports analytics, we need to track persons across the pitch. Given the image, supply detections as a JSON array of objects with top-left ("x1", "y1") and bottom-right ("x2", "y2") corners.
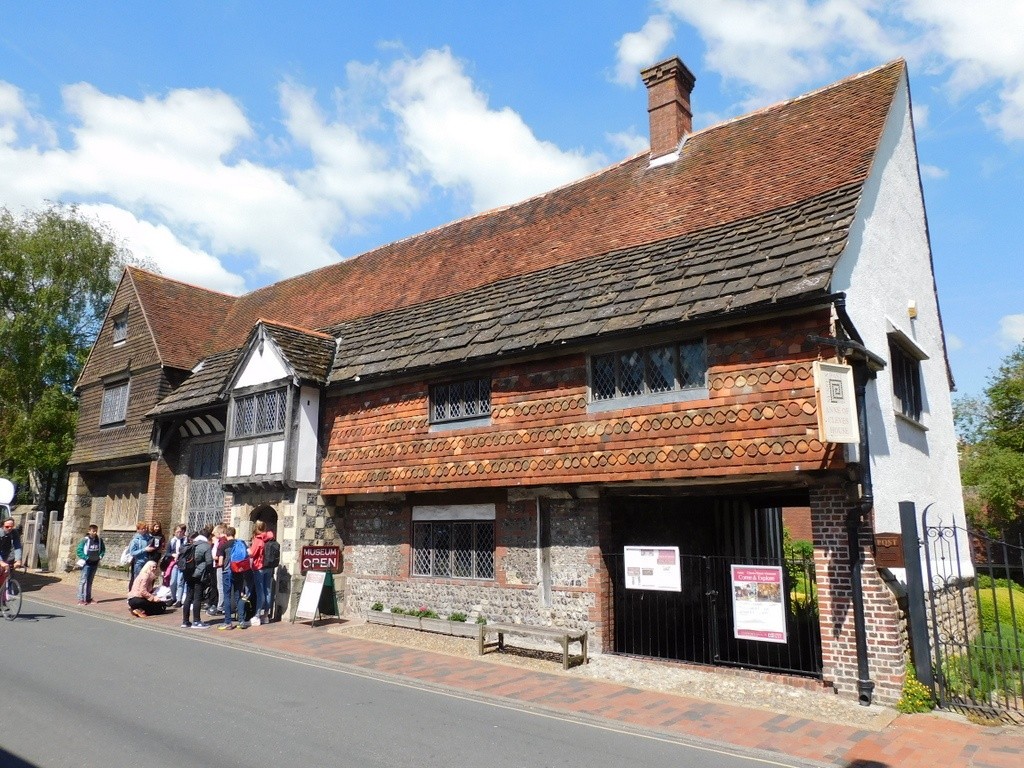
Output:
[
  {"x1": 0, "y1": 518, "x2": 23, "y2": 611},
  {"x1": 76, "y1": 525, "x2": 105, "y2": 606},
  {"x1": 120, "y1": 517, "x2": 281, "y2": 631}
]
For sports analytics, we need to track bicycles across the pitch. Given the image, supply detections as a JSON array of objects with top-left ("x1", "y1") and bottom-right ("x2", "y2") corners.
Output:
[{"x1": 0, "y1": 564, "x2": 27, "y2": 621}]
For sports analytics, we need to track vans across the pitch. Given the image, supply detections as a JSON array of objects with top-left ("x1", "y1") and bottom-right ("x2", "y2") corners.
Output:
[{"x1": 0, "y1": 478, "x2": 14, "y2": 538}]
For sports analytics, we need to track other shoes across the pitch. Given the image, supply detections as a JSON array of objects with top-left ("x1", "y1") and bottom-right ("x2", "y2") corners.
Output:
[
  {"x1": 216, "y1": 623, "x2": 232, "y2": 630},
  {"x1": 78, "y1": 600, "x2": 87, "y2": 605},
  {"x1": 260, "y1": 615, "x2": 269, "y2": 624},
  {"x1": 181, "y1": 622, "x2": 192, "y2": 627},
  {"x1": 200, "y1": 603, "x2": 222, "y2": 615},
  {"x1": 129, "y1": 607, "x2": 147, "y2": 619},
  {"x1": 237, "y1": 621, "x2": 247, "y2": 629},
  {"x1": 247, "y1": 616, "x2": 261, "y2": 626},
  {"x1": 164, "y1": 598, "x2": 182, "y2": 608},
  {"x1": 191, "y1": 622, "x2": 210, "y2": 628},
  {"x1": 86, "y1": 599, "x2": 97, "y2": 604}
]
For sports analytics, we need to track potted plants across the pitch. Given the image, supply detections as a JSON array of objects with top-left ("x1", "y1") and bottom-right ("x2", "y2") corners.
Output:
[{"x1": 366, "y1": 609, "x2": 484, "y2": 639}]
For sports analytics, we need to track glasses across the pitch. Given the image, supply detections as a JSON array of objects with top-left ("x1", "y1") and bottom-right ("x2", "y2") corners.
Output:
[{"x1": 4, "y1": 526, "x2": 13, "y2": 529}]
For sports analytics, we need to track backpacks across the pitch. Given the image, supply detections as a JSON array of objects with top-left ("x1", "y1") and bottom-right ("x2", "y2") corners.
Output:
[
  {"x1": 262, "y1": 540, "x2": 281, "y2": 567},
  {"x1": 176, "y1": 541, "x2": 205, "y2": 571},
  {"x1": 228, "y1": 539, "x2": 252, "y2": 573}
]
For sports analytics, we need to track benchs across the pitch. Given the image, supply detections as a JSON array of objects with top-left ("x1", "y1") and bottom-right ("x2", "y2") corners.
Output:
[{"x1": 477, "y1": 622, "x2": 588, "y2": 671}]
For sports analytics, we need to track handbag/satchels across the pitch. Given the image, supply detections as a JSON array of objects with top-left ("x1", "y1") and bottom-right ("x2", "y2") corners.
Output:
[{"x1": 120, "y1": 536, "x2": 141, "y2": 569}]
